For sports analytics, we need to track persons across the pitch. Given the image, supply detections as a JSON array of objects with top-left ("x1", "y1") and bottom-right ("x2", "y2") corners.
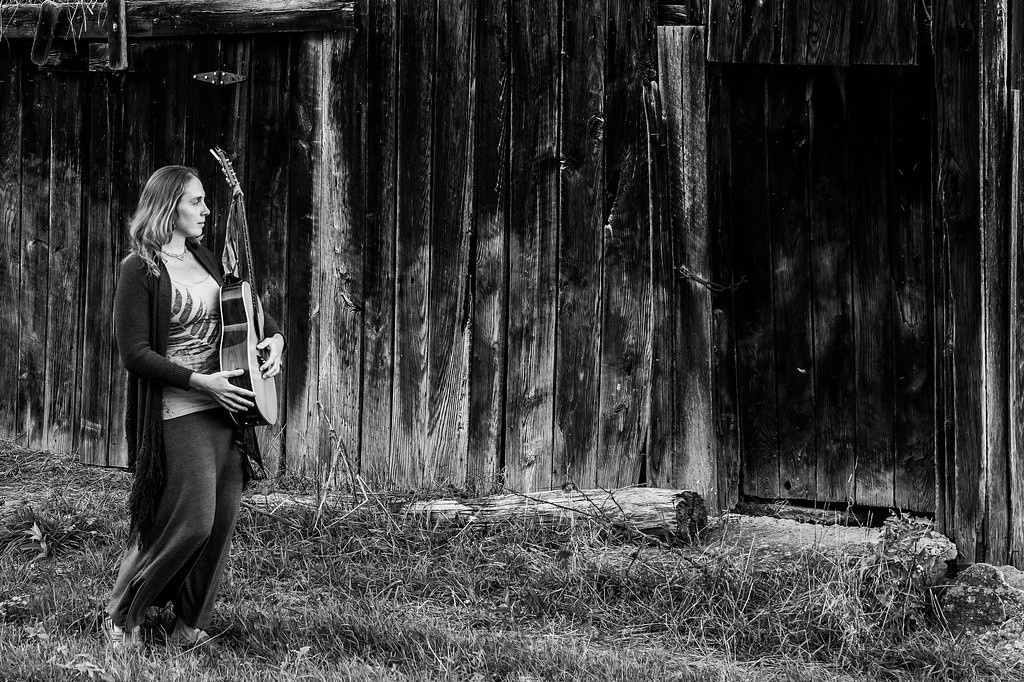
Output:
[{"x1": 99, "y1": 166, "x2": 285, "y2": 659}]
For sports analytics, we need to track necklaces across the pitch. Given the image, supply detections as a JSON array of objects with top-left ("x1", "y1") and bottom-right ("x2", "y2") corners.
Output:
[{"x1": 162, "y1": 245, "x2": 188, "y2": 262}]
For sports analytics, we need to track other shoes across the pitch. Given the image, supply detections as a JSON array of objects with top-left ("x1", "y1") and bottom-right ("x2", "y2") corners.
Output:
[
  {"x1": 168, "y1": 631, "x2": 229, "y2": 665},
  {"x1": 101, "y1": 615, "x2": 144, "y2": 658}
]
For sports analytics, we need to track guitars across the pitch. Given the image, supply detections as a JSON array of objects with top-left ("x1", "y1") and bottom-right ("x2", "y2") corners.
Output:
[{"x1": 209, "y1": 144, "x2": 280, "y2": 429}]
